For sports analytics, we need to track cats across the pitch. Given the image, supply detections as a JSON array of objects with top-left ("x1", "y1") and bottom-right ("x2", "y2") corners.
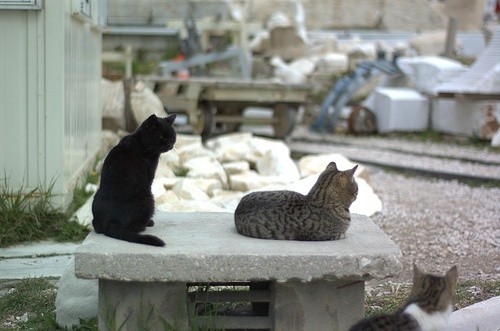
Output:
[
  {"x1": 91, "y1": 113, "x2": 178, "y2": 246},
  {"x1": 345, "y1": 263, "x2": 459, "y2": 331},
  {"x1": 234, "y1": 162, "x2": 360, "y2": 241}
]
